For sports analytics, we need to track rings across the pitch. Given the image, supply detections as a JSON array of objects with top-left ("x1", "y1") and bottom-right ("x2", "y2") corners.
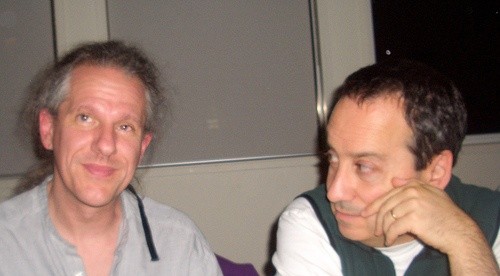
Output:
[{"x1": 391, "y1": 208, "x2": 399, "y2": 220}]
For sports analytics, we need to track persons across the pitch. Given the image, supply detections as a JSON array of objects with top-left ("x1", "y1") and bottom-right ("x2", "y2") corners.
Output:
[
  {"x1": 0, "y1": 40, "x2": 225, "y2": 276},
  {"x1": 271, "y1": 61, "x2": 500, "y2": 274}
]
